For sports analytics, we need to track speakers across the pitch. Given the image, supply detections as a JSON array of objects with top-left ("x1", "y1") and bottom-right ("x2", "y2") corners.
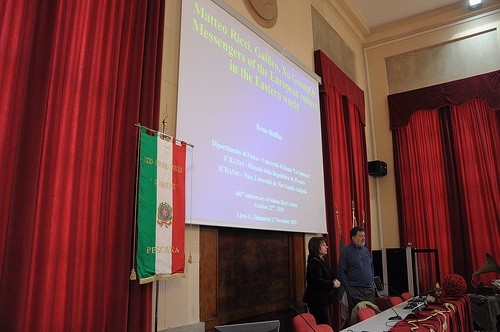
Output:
[{"x1": 368, "y1": 161, "x2": 387, "y2": 176}]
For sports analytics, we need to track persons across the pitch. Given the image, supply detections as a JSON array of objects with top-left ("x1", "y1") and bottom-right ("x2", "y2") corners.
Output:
[{"x1": 303, "y1": 227, "x2": 375, "y2": 326}]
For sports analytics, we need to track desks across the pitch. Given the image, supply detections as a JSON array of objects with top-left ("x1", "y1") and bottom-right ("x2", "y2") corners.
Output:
[{"x1": 339, "y1": 294, "x2": 469, "y2": 332}]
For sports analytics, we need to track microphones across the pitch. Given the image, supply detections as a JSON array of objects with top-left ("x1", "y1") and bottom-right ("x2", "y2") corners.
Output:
[
  {"x1": 378, "y1": 289, "x2": 402, "y2": 320},
  {"x1": 287, "y1": 303, "x2": 316, "y2": 332},
  {"x1": 389, "y1": 286, "x2": 414, "y2": 309}
]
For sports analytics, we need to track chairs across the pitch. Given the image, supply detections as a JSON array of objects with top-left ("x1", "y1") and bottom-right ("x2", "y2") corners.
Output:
[{"x1": 292, "y1": 293, "x2": 412, "y2": 332}]
